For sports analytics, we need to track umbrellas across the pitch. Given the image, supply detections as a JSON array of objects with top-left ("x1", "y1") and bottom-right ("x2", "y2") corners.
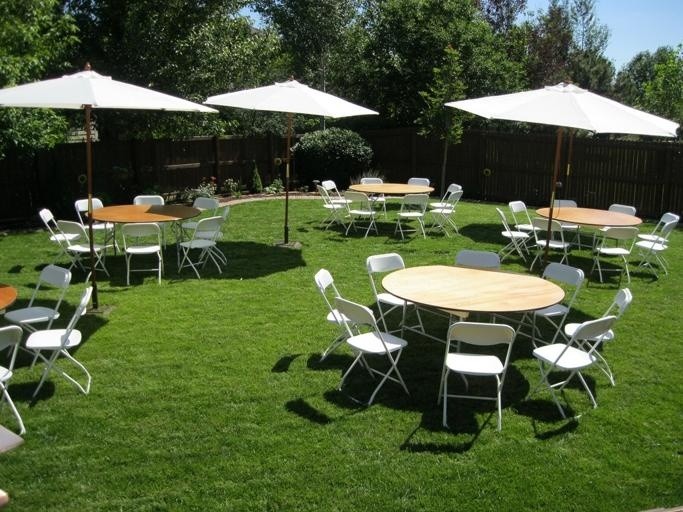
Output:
[
  {"x1": 203, "y1": 77, "x2": 379, "y2": 245},
  {"x1": 444, "y1": 78, "x2": 679, "y2": 272},
  {"x1": 0, "y1": 62, "x2": 220, "y2": 310}
]
